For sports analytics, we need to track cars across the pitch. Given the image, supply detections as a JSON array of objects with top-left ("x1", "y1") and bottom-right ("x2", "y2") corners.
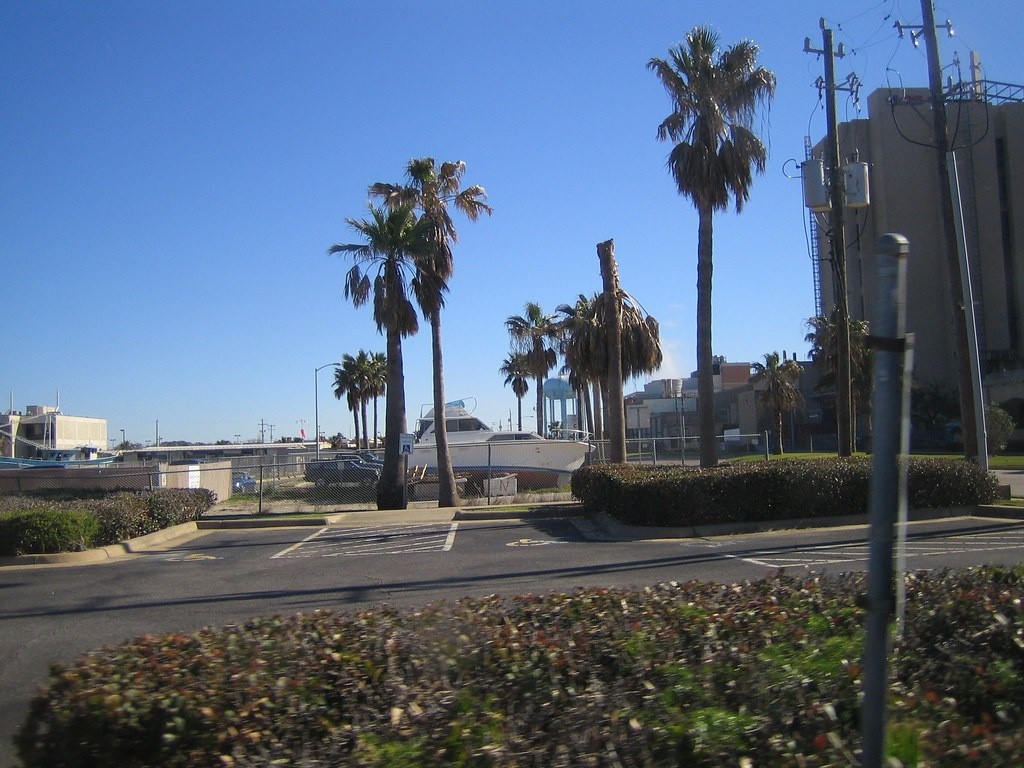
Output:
[{"x1": 232, "y1": 470, "x2": 257, "y2": 494}]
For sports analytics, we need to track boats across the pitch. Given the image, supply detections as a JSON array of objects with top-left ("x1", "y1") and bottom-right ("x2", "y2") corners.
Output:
[{"x1": 413, "y1": 395, "x2": 598, "y2": 492}]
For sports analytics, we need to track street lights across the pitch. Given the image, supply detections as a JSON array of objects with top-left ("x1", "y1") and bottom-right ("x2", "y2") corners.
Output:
[
  {"x1": 120, "y1": 429, "x2": 126, "y2": 443},
  {"x1": 315, "y1": 361, "x2": 342, "y2": 459},
  {"x1": 235, "y1": 434, "x2": 241, "y2": 443}
]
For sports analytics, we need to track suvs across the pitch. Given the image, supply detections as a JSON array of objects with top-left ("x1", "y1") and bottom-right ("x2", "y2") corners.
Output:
[{"x1": 303, "y1": 451, "x2": 386, "y2": 487}]
